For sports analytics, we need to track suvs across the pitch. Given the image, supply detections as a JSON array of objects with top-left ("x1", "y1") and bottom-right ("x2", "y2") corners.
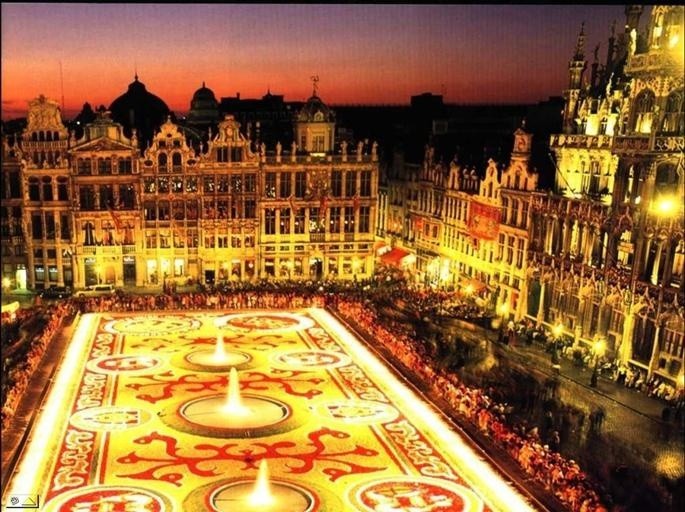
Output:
[{"x1": 72, "y1": 284, "x2": 116, "y2": 299}]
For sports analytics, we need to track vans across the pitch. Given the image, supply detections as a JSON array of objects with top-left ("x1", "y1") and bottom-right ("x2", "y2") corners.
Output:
[{"x1": 39, "y1": 286, "x2": 72, "y2": 299}]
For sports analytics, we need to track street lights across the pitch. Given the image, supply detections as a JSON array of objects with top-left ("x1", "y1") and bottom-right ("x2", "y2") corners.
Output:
[
  {"x1": 551, "y1": 319, "x2": 564, "y2": 364},
  {"x1": 590, "y1": 335, "x2": 607, "y2": 384}
]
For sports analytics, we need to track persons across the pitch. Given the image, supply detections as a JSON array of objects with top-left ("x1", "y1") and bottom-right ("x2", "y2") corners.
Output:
[
  {"x1": 336, "y1": 275, "x2": 685, "y2": 512},
  {"x1": 73, "y1": 277, "x2": 352, "y2": 311},
  {"x1": 1, "y1": 294, "x2": 73, "y2": 433}
]
